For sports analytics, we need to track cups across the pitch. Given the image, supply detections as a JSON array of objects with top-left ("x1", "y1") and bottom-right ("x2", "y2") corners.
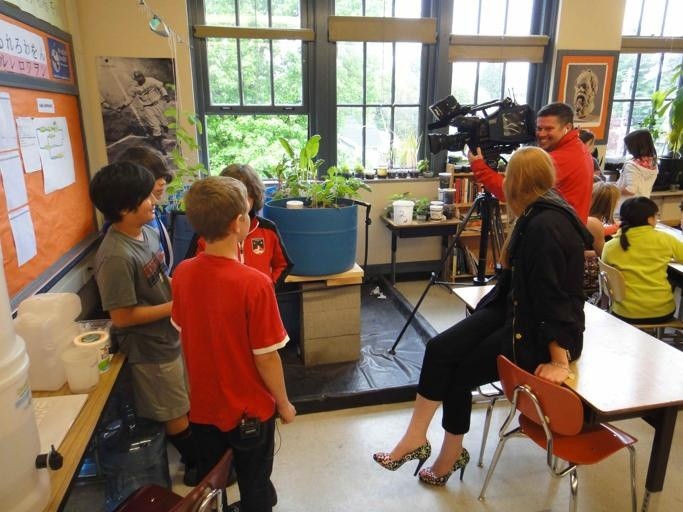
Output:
[
  {"x1": 430, "y1": 200, "x2": 444, "y2": 220},
  {"x1": 393, "y1": 200, "x2": 414, "y2": 226}
]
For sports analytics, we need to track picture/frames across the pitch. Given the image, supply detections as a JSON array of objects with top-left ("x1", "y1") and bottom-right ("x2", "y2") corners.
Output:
[{"x1": 551, "y1": 48, "x2": 620, "y2": 146}]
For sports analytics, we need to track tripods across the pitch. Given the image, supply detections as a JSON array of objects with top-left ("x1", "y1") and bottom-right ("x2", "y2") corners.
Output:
[{"x1": 388, "y1": 196, "x2": 505, "y2": 355}]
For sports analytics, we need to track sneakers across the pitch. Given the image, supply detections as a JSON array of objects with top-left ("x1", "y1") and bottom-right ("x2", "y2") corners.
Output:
[{"x1": 183, "y1": 464, "x2": 237, "y2": 488}]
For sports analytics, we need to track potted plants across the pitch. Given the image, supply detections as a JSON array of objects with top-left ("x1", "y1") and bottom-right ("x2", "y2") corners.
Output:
[
  {"x1": 157, "y1": 76, "x2": 373, "y2": 278},
  {"x1": 638, "y1": 59, "x2": 682, "y2": 191}
]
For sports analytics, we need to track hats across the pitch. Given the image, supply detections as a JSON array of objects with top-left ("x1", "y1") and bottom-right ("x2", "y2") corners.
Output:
[{"x1": 113, "y1": 146, "x2": 175, "y2": 185}]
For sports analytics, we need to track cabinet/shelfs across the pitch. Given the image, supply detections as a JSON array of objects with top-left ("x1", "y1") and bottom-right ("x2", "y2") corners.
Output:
[{"x1": 447, "y1": 163, "x2": 513, "y2": 286}]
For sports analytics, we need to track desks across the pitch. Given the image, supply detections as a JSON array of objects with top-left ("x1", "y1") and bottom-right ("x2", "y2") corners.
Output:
[
  {"x1": 22, "y1": 320, "x2": 127, "y2": 511},
  {"x1": 451, "y1": 285, "x2": 682, "y2": 512},
  {"x1": 380, "y1": 214, "x2": 464, "y2": 289},
  {"x1": 606, "y1": 216, "x2": 683, "y2": 273},
  {"x1": 283, "y1": 261, "x2": 367, "y2": 366}
]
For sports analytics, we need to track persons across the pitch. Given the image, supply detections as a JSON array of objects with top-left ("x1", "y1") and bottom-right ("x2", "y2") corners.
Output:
[
  {"x1": 101, "y1": 68, "x2": 170, "y2": 155},
  {"x1": 567, "y1": 68, "x2": 600, "y2": 123}
]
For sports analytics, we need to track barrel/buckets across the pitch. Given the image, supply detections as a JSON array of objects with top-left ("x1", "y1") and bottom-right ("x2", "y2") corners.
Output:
[
  {"x1": 14, "y1": 291, "x2": 81, "y2": 390},
  {"x1": 73, "y1": 330, "x2": 112, "y2": 374},
  {"x1": 170, "y1": 210, "x2": 197, "y2": 274},
  {"x1": 393, "y1": 200, "x2": 415, "y2": 223},
  {"x1": 1, "y1": 334, "x2": 65, "y2": 511},
  {"x1": 266, "y1": 193, "x2": 357, "y2": 278}
]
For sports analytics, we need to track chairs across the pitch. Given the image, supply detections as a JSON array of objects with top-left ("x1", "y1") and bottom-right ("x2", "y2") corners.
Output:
[
  {"x1": 474, "y1": 382, "x2": 508, "y2": 466},
  {"x1": 114, "y1": 447, "x2": 234, "y2": 512},
  {"x1": 595, "y1": 256, "x2": 682, "y2": 345},
  {"x1": 479, "y1": 355, "x2": 638, "y2": 511}
]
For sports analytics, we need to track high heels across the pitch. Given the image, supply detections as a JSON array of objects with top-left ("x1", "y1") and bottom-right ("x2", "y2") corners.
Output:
[
  {"x1": 372, "y1": 438, "x2": 432, "y2": 477},
  {"x1": 419, "y1": 447, "x2": 471, "y2": 487}
]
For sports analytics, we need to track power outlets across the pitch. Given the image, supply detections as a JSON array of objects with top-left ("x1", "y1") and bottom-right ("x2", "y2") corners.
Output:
[{"x1": 80, "y1": 261, "x2": 96, "y2": 283}]
